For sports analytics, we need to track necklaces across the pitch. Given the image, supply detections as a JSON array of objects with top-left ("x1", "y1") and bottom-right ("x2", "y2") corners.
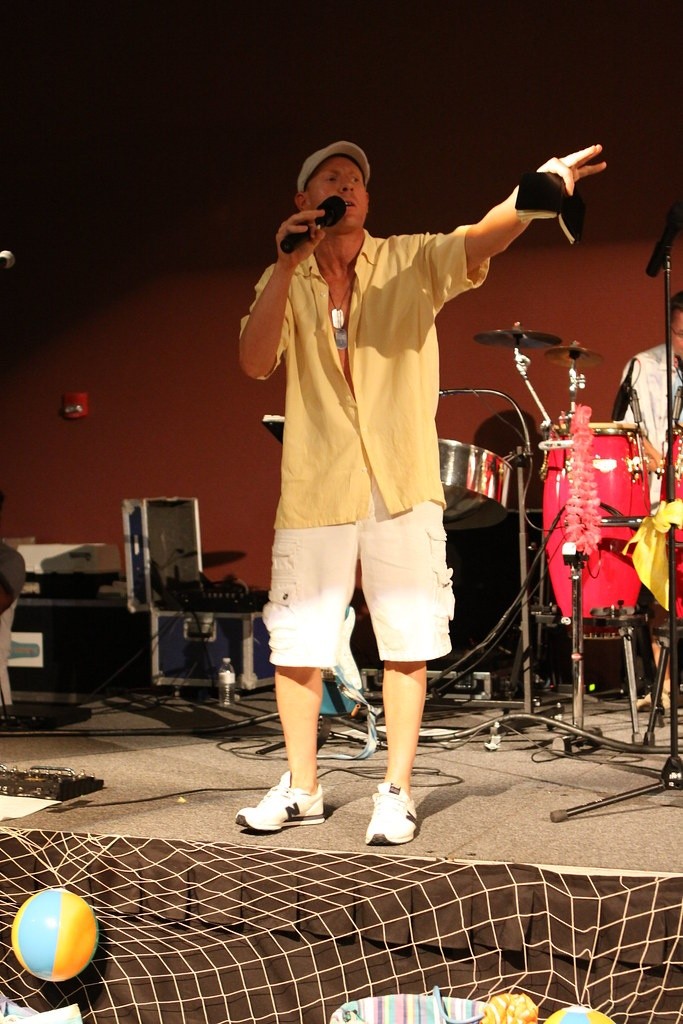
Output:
[{"x1": 327, "y1": 272, "x2": 355, "y2": 350}]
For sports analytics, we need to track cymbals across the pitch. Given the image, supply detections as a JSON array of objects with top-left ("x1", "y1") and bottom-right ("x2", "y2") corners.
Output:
[
  {"x1": 472, "y1": 327, "x2": 562, "y2": 348},
  {"x1": 544, "y1": 346, "x2": 604, "y2": 368}
]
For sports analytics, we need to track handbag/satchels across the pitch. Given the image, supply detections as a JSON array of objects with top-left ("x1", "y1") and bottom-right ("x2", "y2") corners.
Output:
[{"x1": 329, "y1": 985, "x2": 487, "y2": 1024}]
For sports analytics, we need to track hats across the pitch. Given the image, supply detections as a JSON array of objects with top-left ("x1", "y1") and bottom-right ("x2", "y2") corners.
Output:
[{"x1": 298, "y1": 140, "x2": 370, "y2": 191}]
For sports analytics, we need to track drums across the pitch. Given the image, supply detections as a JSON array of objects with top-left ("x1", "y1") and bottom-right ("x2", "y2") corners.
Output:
[
  {"x1": 439, "y1": 438, "x2": 512, "y2": 530},
  {"x1": 541, "y1": 423, "x2": 652, "y2": 640},
  {"x1": 659, "y1": 427, "x2": 683, "y2": 628}
]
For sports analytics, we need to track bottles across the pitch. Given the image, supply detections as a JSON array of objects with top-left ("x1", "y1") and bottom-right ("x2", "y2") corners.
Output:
[{"x1": 218, "y1": 657, "x2": 235, "y2": 708}]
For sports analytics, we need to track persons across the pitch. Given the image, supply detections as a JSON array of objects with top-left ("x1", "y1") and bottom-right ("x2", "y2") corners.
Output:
[
  {"x1": 610, "y1": 292, "x2": 683, "y2": 713},
  {"x1": 234, "y1": 140, "x2": 606, "y2": 846}
]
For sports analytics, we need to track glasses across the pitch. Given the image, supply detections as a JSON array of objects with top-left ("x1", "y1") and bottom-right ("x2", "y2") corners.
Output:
[{"x1": 671, "y1": 326, "x2": 683, "y2": 337}]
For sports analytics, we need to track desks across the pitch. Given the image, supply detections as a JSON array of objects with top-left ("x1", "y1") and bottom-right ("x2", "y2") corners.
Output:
[{"x1": 13, "y1": 598, "x2": 127, "y2": 612}]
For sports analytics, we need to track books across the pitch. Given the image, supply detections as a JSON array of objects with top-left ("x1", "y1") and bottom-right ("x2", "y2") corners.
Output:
[{"x1": 515, "y1": 169, "x2": 586, "y2": 244}]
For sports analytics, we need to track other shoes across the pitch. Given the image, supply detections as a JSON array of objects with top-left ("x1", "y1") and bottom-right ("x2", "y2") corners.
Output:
[{"x1": 637, "y1": 679, "x2": 670, "y2": 711}]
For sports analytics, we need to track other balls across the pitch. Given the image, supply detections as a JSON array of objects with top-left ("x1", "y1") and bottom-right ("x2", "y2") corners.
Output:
[
  {"x1": 11, "y1": 888, "x2": 99, "y2": 982},
  {"x1": 543, "y1": 1006, "x2": 616, "y2": 1024}
]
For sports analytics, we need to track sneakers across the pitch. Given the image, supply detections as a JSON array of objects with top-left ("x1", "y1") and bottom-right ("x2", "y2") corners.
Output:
[
  {"x1": 235, "y1": 770, "x2": 324, "y2": 832},
  {"x1": 365, "y1": 781, "x2": 418, "y2": 843}
]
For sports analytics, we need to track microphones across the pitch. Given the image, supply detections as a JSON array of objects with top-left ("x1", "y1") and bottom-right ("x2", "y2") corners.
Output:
[
  {"x1": 611, "y1": 363, "x2": 634, "y2": 421},
  {"x1": 646, "y1": 201, "x2": 683, "y2": 277},
  {"x1": 280, "y1": 196, "x2": 347, "y2": 254}
]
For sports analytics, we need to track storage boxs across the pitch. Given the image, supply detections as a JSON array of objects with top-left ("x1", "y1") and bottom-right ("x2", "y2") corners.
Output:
[{"x1": 122, "y1": 494, "x2": 276, "y2": 691}]
